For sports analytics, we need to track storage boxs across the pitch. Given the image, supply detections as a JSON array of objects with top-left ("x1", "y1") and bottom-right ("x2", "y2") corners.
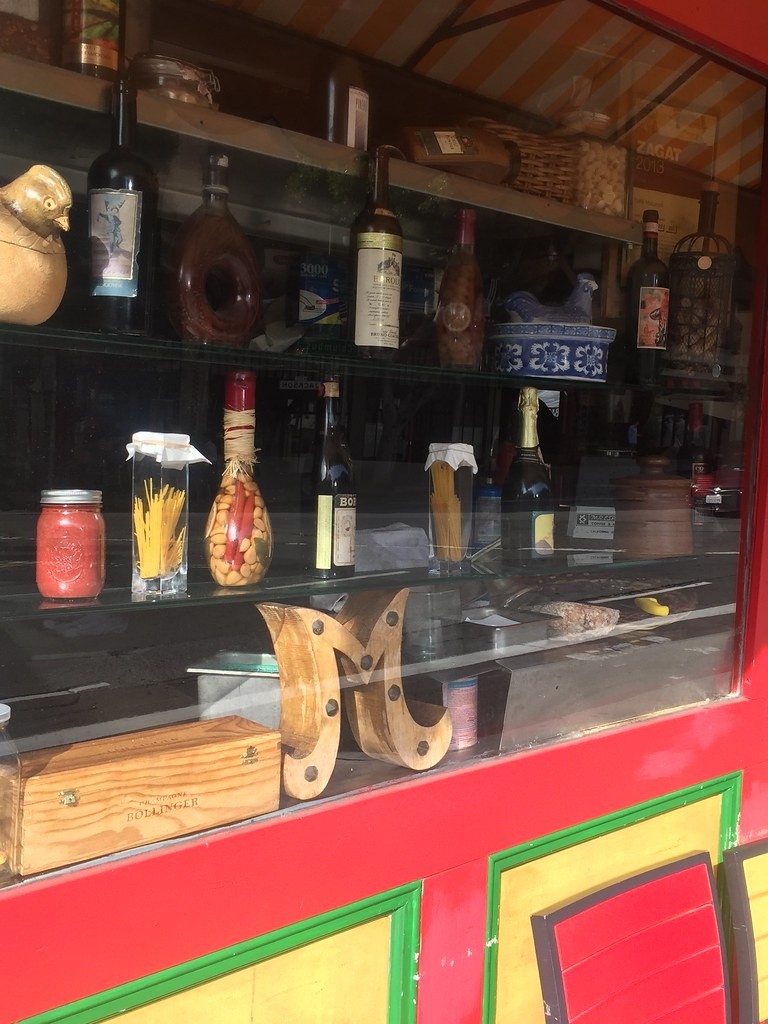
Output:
[{"x1": 0, "y1": 715, "x2": 282, "y2": 877}]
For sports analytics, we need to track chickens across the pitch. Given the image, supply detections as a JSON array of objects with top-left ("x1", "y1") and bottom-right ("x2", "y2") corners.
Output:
[
  {"x1": 503, "y1": 272, "x2": 599, "y2": 325},
  {"x1": 0, "y1": 164, "x2": 73, "y2": 327}
]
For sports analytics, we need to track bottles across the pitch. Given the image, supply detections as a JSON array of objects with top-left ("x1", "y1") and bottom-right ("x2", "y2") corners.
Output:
[
  {"x1": 318, "y1": 55, "x2": 370, "y2": 152},
  {"x1": 88, "y1": 76, "x2": 160, "y2": 337},
  {"x1": 344, "y1": 143, "x2": 404, "y2": 364},
  {"x1": 499, "y1": 385, "x2": 556, "y2": 561},
  {"x1": 57, "y1": 0, "x2": 125, "y2": 84},
  {"x1": 674, "y1": 400, "x2": 716, "y2": 526},
  {"x1": 302, "y1": 370, "x2": 358, "y2": 579},
  {"x1": 202, "y1": 366, "x2": 272, "y2": 587},
  {"x1": 435, "y1": 205, "x2": 485, "y2": 371},
  {"x1": 620, "y1": 208, "x2": 670, "y2": 384},
  {"x1": 164, "y1": 153, "x2": 262, "y2": 350},
  {"x1": 393, "y1": 122, "x2": 522, "y2": 186}
]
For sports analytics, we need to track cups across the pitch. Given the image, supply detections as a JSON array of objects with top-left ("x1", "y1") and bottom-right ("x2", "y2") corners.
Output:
[
  {"x1": 443, "y1": 675, "x2": 478, "y2": 750},
  {"x1": 129, "y1": 433, "x2": 189, "y2": 598},
  {"x1": 427, "y1": 443, "x2": 474, "y2": 573},
  {"x1": 402, "y1": 585, "x2": 462, "y2": 657}
]
